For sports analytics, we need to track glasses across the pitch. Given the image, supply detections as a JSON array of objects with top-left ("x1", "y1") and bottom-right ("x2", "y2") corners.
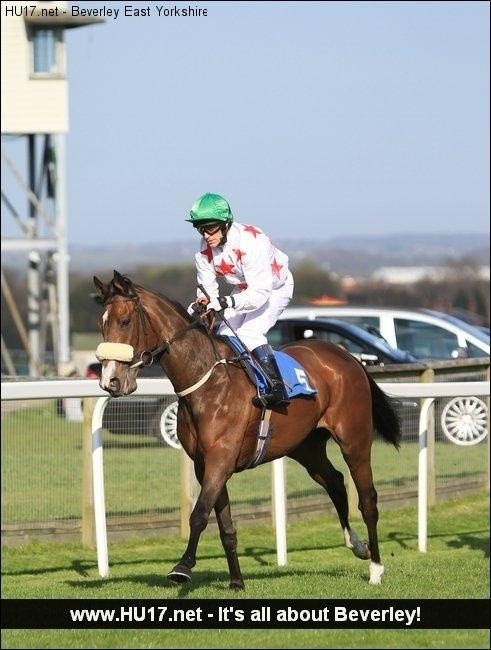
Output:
[{"x1": 196, "y1": 222, "x2": 225, "y2": 236}]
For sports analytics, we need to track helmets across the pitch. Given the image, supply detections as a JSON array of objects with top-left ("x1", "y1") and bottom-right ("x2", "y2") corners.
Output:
[{"x1": 184, "y1": 191, "x2": 235, "y2": 224}]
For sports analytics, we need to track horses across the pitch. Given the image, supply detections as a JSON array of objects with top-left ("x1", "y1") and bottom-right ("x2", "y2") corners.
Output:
[{"x1": 93, "y1": 270, "x2": 404, "y2": 590}]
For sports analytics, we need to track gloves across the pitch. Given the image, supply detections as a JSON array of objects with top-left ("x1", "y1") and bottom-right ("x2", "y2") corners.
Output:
[{"x1": 205, "y1": 293, "x2": 236, "y2": 312}]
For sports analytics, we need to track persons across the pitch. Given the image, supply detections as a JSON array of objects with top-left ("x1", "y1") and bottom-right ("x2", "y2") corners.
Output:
[{"x1": 185, "y1": 193, "x2": 296, "y2": 409}]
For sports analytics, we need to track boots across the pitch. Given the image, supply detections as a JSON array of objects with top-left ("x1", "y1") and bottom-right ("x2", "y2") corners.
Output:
[{"x1": 250, "y1": 354, "x2": 292, "y2": 411}]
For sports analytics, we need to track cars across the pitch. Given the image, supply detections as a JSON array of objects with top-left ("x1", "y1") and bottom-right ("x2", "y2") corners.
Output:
[
  {"x1": 83, "y1": 318, "x2": 490, "y2": 450},
  {"x1": 278, "y1": 305, "x2": 491, "y2": 363}
]
